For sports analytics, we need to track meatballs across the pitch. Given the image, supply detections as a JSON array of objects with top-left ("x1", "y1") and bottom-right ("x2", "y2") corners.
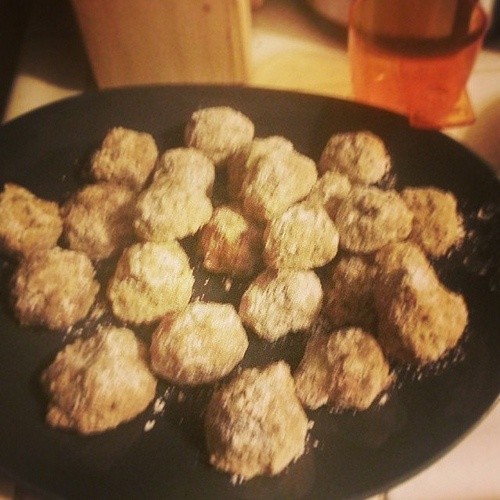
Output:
[{"x1": 0, "y1": 105, "x2": 468, "y2": 481}]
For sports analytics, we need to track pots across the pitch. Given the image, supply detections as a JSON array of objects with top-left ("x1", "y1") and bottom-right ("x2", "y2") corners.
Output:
[{"x1": 1, "y1": 82, "x2": 500, "y2": 500}]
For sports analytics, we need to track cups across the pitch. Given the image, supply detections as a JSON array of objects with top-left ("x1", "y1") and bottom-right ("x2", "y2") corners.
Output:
[{"x1": 346, "y1": 1, "x2": 491, "y2": 137}]
